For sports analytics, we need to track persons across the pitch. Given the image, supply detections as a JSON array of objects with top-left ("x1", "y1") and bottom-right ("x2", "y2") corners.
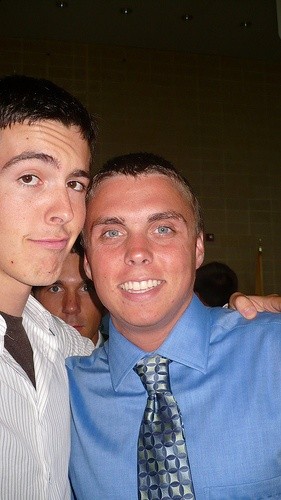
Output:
[
  {"x1": 33, "y1": 151, "x2": 280, "y2": 500},
  {"x1": 0, "y1": 75, "x2": 281, "y2": 500}
]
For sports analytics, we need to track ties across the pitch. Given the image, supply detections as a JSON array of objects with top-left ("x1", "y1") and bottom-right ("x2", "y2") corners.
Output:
[{"x1": 133, "y1": 355, "x2": 197, "y2": 500}]
